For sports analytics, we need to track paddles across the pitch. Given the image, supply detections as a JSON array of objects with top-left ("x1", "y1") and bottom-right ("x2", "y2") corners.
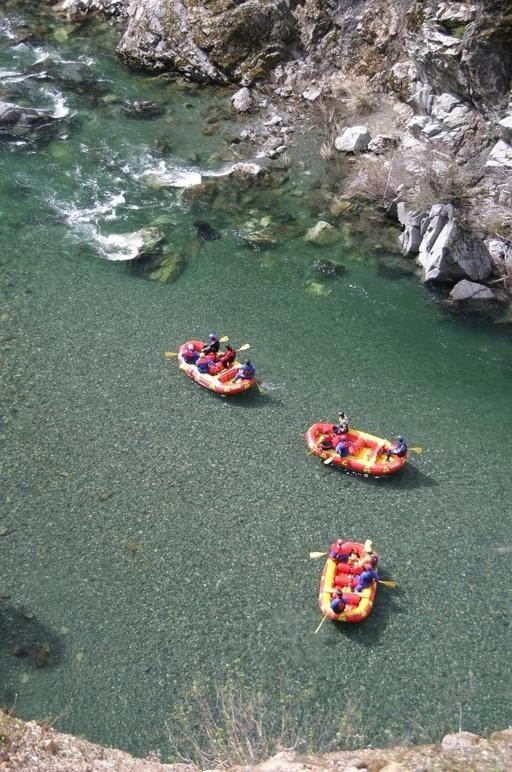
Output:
[
  {"x1": 219, "y1": 336, "x2": 229, "y2": 343},
  {"x1": 315, "y1": 610, "x2": 330, "y2": 634},
  {"x1": 376, "y1": 580, "x2": 395, "y2": 589},
  {"x1": 408, "y1": 447, "x2": 422, "y2": 456},
  {"x1": 254, "y1": 380, "x2": 266, "y2": 395},
  {"x1": 359, "y1": 539, "x2": 372, "y2": 557},
  {"x1": 324, "y1": 587, "x2": 337, "y2": 593},
  {"x1": 323, "y1": 455, "x2": 339, "y2": 465},
  {"x1": 165, "y1": 352, "x2": 180, "y2": 358},
  {"x1": 309, "y1": 551, "x2": 326, "y2": 559},
  {"x1": 234, "y1": 344, "x2": 250, "y2": 353}
]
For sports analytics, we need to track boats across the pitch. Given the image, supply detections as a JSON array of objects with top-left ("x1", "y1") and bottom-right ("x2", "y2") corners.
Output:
[
  {"x1": 303, "y1": 420, "x2": 409, "y2": 476},
  {"x1": 176, "y1": 336, "x2": 257, "y2": 395},
  {"x1": 317, "y1": 539, "x2": 380, "y2": 624}
]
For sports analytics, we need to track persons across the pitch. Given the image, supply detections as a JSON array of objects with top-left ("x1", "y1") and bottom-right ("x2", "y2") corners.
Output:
[
  {"x1": 384, "y1": 434, "x2": 407, "y2": 461},
  {"x1": 328, "y1": 411, "x2": 352, "y2": 436},
  {"x1": 328, "y1": 538, "x2": 379, "y2": 614},
  {"x1": 329, "y1": 432, "x2": 353, "y2": 457},
  {"x1": 181, "y1": 332, "x2": 236, "y2": 374},
  {"x1": 232, "y1": 358, "x2": 258, "y2": 384}
]
